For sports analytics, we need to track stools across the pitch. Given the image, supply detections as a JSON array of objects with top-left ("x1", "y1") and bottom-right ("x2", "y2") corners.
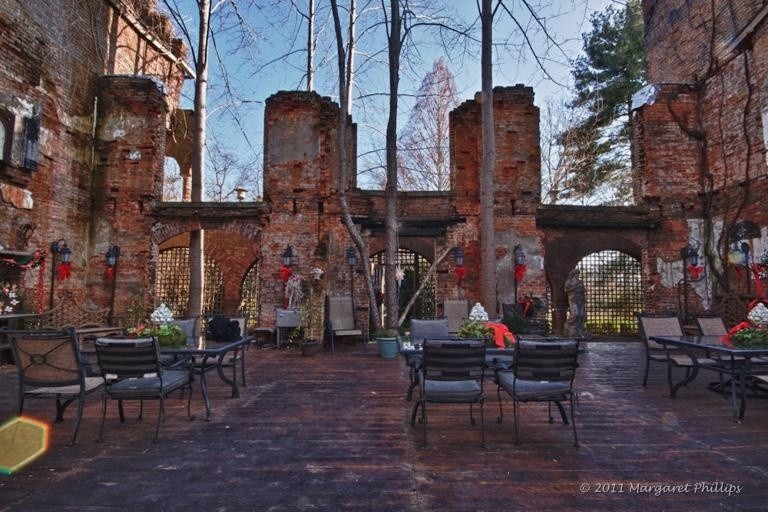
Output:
[{"x1": 254, "y1": 327, "x2": 276, "y2": 347}]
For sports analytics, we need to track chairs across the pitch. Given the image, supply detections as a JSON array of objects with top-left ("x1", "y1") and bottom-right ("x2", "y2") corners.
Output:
[
  {"x1": 496, "y1": 338, "x2": 581, "y2": 450},
  {"x1": 638, "y1": 317, "x2": 717, "y2": 392},
  {"x1": 411, "y1": 338, "x2": 486, "y2": 447},
  {"x1": 11, "y1": 315, "x2": 251, "y2": 447},
  {"x1": 697, "y1": 317, "x2": 762, "y2": 400},
  {"x1": 737, "y1": 373, "x2": 768, "y2": 419},
  {"x1": 409, "y1": 320, "x2": 449, "y2": 407},
  {"x1": 328, "y1": 296, "x2": 365, "y2": 354},
  {"x1": 444, "y1": 299, "x2": 473, "y2": 339}
]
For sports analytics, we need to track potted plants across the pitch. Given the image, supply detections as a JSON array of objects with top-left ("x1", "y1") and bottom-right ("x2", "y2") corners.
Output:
[
  {"x1": 287, "y1": 296, "x2": 324, "y2": 357},
  {"x1": 376, "y1": 328, "x2": 400, "y2": 360}
]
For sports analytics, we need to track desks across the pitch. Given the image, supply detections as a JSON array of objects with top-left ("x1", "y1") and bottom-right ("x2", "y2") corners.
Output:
[
  {"x1": 399, "y1": 346, "x2": 570, "y2": 427},
  {"x1": 648, "y1": 336, "x2": 768, "y2": 423}
]
